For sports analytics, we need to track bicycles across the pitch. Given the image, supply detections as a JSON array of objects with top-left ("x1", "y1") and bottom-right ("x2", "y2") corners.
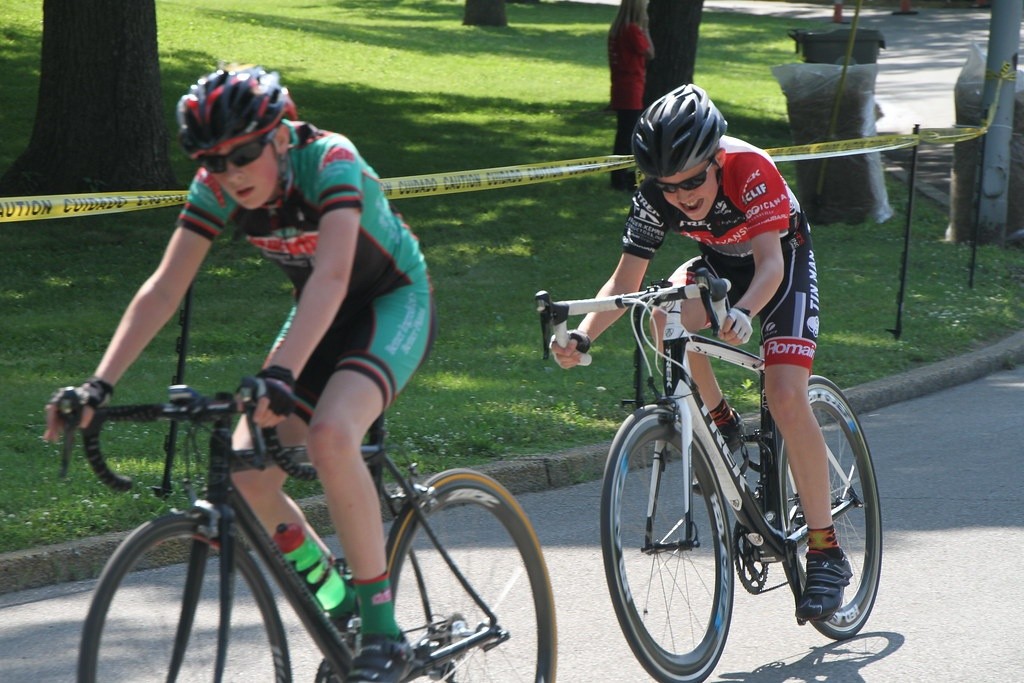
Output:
[
  {"x1": 51, "y1": 376, "x2": 559, "y2": 683},
  {"x1": 535, "y1": 266, "x2": 883, "y2": 682}
]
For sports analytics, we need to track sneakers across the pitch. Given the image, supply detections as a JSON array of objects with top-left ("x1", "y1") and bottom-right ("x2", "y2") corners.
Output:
[
  {"x1": 795, "y1": 546, "x2": 853, "y2": 621},
  {"x1": 316, "y1": 613, "x2": 417, "y2": 683},
  {"x1": 688, "y1": 407, "x2": 747, "y2": 494}
]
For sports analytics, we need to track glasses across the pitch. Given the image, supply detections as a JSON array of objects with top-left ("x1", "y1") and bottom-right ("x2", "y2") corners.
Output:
[
  {"x1": 190, "y1": 128, "x2": 277, "y2": 173},
  {"x1": 649, "y1": 161, "x2": 713, "y2": 193}
]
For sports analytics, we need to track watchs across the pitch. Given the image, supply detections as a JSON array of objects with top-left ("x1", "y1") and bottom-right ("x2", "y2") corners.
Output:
[
  {"x1": 255, "y1": 366, "x2": 294, "y2": 384},
  {"x1": 734, "y1": 306, "x2": 751, "y2": 316}
]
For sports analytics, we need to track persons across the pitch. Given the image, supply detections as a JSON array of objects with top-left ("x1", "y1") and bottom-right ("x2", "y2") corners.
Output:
[
  {"x1": 609, "y1": 0, "x2": 654, "y2": 190},
  {"x1": 548, "y1": 84, "x2": 852, "y2": 622},
  {"x1": 42, "y1": 62, "x2": 438, "y2": 683}
]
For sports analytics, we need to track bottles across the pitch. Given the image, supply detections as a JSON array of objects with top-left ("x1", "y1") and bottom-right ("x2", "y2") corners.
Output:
[{"x1": 275, "y1": 524, "x2": 356, "y2": 618}]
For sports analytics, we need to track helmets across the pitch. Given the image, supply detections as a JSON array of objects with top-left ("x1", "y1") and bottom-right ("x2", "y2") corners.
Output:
[
  {"x1": 176, "y1": 65, "x2": 297, "y2": 160},
  {"x1": 631, "y1": 84, "x2": 728, "y2": 178}
]
